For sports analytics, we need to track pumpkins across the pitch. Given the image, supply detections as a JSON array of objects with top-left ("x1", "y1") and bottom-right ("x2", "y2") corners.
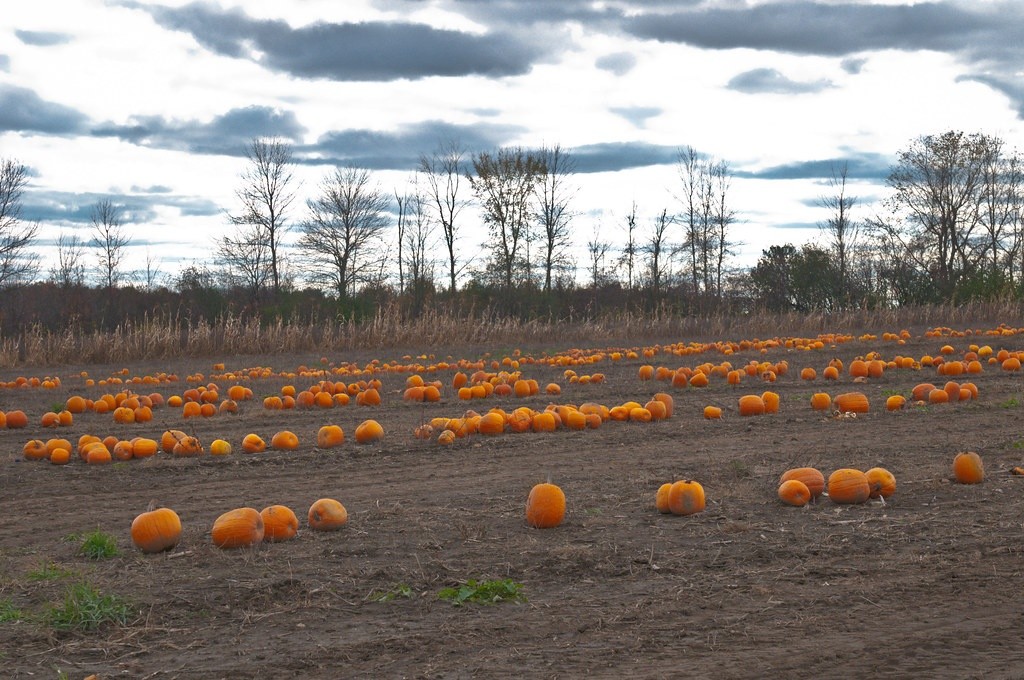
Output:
[{"x1": 0, "y1": 323, "x2": 1024, "y2": 552}]
